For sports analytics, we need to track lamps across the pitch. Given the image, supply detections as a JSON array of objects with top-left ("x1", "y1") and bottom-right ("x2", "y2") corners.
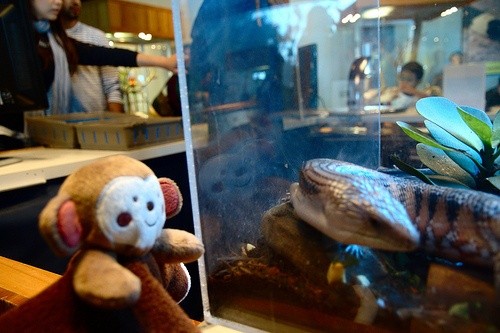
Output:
[{"x1": 348, "y1": 56, "x2": 375, "y2": 81}]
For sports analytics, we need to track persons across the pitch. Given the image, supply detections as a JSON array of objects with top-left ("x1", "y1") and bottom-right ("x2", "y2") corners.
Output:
[
  {"x1": 381, "y1": 60, "x2": 430, "y2": 114},
  {"x1": 0, "y1": 0, "x2": 189, "y2": 150}
]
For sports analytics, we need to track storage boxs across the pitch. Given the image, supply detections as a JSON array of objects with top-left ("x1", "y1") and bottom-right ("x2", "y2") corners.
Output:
[{"x1": 26, "y1": 111, "x2": 183, "y2": 151}]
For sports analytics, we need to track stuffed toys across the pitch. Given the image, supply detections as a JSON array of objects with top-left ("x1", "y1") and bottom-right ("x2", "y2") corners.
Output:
[{"x1": 39, "y1": 151, "x2": 206, "y2": 333}]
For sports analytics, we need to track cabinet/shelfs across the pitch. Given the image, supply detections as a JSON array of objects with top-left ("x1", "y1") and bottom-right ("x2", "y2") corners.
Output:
[{"x1": 80, "y1": 0, "x2": 186, "y2": 46}]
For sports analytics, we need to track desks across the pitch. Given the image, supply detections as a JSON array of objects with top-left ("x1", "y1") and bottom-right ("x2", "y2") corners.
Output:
[
  {"x1": 311, "y1": 107, "x2": 428, "y2": 153},
  {"x1": 328, "y1": 104, "x2": 425, "y2": 126}
]
